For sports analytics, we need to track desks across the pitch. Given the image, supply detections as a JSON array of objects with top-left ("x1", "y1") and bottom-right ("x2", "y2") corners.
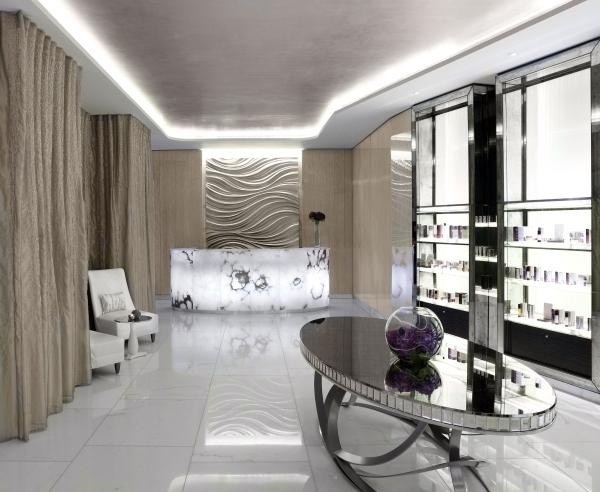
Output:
[{"x1": 299, "y1": 314, "x2": 557, "y2": 492}]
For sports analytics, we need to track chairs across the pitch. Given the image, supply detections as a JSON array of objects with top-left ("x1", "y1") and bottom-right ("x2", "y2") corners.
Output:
[
  {"x1": 89, "y1": 329, "x2": 126, "y2": 379},
  {"x1": 86, "y1": 267, "x2": 159, "y2": 350}
]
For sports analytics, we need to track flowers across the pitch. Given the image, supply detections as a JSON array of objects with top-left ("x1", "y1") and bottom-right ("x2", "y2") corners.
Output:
[{"x1": 309, "y1": 210, "x2": 326, "y2": 224}]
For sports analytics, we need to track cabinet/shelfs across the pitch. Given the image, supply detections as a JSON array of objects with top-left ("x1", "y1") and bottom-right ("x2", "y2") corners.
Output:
[
  {"x1": 498, "y1": 206, "x2": 600, "y2": 393},
  {"x1": 412, "y1": 210, "x2": 475, "y2": 343}
]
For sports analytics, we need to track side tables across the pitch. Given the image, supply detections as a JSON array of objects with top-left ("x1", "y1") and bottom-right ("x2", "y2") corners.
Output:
[{"x1": 115, "y1": 315, "x2": 153, "y2": 361}]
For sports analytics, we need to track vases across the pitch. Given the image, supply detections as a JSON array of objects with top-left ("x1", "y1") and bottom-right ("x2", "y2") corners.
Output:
[{"x1": 314, "y1": 222, "x2": 320, "y2": 247}]
